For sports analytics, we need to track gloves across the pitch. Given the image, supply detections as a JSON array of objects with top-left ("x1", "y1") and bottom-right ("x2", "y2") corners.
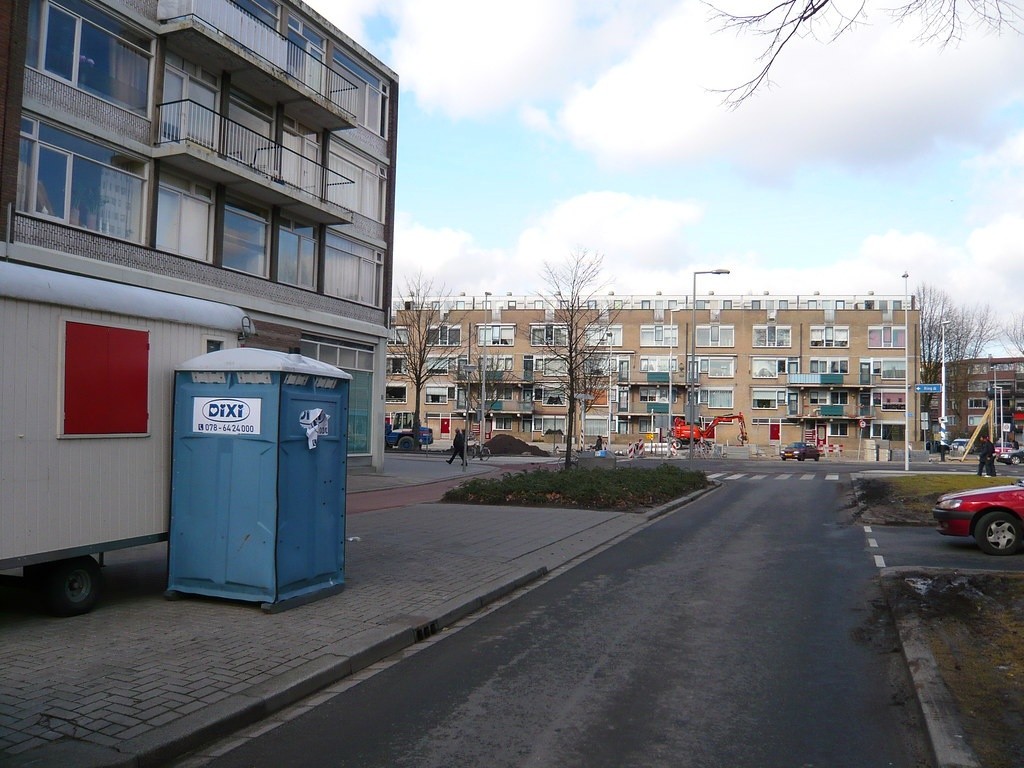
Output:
[
  {"x1": 992, "y1": 453, "x2": 997, "y2": 457},
  {"x1": 977, "y1": 455, "x2": 981, "y2": 461}
]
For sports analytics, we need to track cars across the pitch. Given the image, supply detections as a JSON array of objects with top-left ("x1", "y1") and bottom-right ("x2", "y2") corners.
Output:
[
  {"x1": 951, "y1": 439, "x2": 974, "y2": 453},
  {"x1": 779, "y1": 442, "x2": 820, "y2": 461},
  {"x1": 993, "y1": 442, "x2": 1012, "y2": 453},
  {"x1": 996, "y1": 449, "x2": 1024, "y2": 465},
  {"x1": 933, "y1": 480, "x2": 1024, "y2": 556}
]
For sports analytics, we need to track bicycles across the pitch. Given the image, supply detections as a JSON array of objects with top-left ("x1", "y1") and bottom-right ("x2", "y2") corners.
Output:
[
  {"x1": 467, "y1": 442, "x2": 490, "y2": 461},
  {"x1": 558, "y1": 449, "x2": 579, "y2": 470}
]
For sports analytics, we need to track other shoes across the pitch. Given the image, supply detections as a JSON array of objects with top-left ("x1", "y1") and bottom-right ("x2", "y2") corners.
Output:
[
  {"x1": 461, "y1": 463, "x2": 467, "y2": 466},
  {"x1": 446, "y1": 460, "x2": 451, "y2": 464},
  {"x1": 983, "y1": 475, "x2": 991, "y2": 477}
]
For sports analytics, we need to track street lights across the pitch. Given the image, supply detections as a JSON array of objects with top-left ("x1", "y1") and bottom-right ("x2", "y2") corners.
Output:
[
  {"x1": 941, "y1": 321, "x2": 951, "y2": 462},
  {"x1": 482, "y1": 292, "x2": 493, "y2": 449},
  {"x1": 668, "y1": 309, "x2": 681, "y2": 430},
  {"x1": 463, "y1": 364, "x2": 477, "y2": 472},
  {"x1": 902, "y1": 271, "x2": 909, "y2": 471},
  {"x1": 992, "y1": 387, "x2": 1003, "y2": 453},
  {"x1": 690, "y1": 269, "x2": 730, "y2": 472},
  {"x1": 606, "y1": 331, "x2": 613, "y2": 452},
  {"x1": 990, "y1": 365, "x2": 1000, "y2": 438}
]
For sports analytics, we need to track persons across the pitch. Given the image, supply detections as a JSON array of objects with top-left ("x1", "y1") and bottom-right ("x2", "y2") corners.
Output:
[
  {"x1": 603, "y1": 441, "x2": 607, "y2": 450},
  {"x1": 445, "y1": 427, "x2": 468, "y2": 466},
  {"x1": 594, "y1": 435, "x2": 602, "y2": 451},
  {"x1": 976, "y1": 435, "x2": 996, "y2": 477}
]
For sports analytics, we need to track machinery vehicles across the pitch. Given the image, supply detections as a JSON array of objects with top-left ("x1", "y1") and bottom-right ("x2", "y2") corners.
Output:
[
  {"x1": 671, "y1": 412, "x2": 749, "y2": 450},
  {"x1": 385, "y1": 411, "x2": 433, "y2": 451}
]
